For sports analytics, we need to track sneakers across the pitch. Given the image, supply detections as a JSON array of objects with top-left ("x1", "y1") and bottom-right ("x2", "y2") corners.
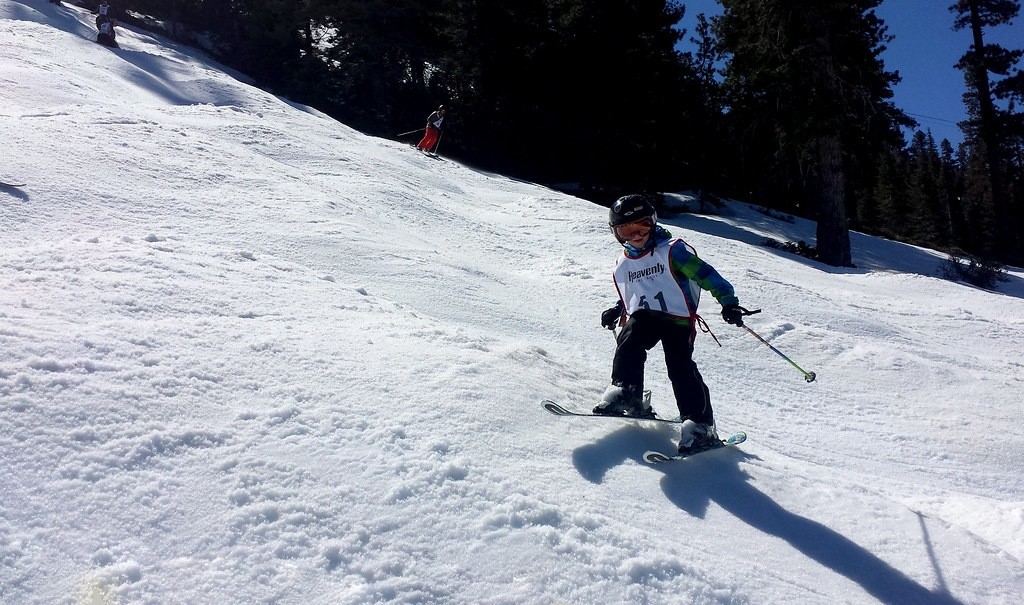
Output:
[
  {"x1": 424, "y1": 148, "x2": 429, "y2": 154},
  {"x1": 678, "y1": 420, "x2": 720, "y2": 454},
  {"x1": 593, "y1": 384, "x2": 644, "y2": 416},
  {"x1": 416, "y1": 145, "x2": 422, "y2": 152}
]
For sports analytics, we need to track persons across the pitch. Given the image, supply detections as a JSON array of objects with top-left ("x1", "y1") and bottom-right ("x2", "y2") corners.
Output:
[
  {"x1": 416, "y1": 104, "x2": 448, "y2": 152},
  {"x1": 593, "y1": 192, "x2": 743, "y2": 453},
  {"x1": 90, "y1": 0, "x2": 115, "y2": 43}
]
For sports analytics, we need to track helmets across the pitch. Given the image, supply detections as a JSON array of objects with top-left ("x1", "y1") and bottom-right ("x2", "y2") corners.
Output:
[
  {"x1": 609, "y1": 195, "x2": 658, "y2": 227},
  {"x1": 438, "y1": 105, "x2": 448, "y2": 111}
]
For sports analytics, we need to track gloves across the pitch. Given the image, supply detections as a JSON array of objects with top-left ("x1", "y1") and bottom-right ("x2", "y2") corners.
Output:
[
  {"x1": 600, "y1": 301, "x2": 622, "y2": 329},
  {"x1": 722, "y1": 306, "x2": 745, "y2": 327}
]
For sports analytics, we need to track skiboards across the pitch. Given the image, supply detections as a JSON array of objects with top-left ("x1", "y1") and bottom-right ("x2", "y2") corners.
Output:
[
  {"x1": 540, "y1": 398, "x2": 747, "y2": 464},
  {"x1": 411, "y1": 145, "x2": 444, "y2": 161}
]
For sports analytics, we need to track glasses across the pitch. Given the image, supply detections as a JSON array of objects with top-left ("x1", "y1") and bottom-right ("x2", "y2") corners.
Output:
[
  {"x1": 614, "y1": 217, "x2": 653, "y2": 239},
  {"x1": 441, "y1": 109, "x2": 446, "y2": 112}
]
